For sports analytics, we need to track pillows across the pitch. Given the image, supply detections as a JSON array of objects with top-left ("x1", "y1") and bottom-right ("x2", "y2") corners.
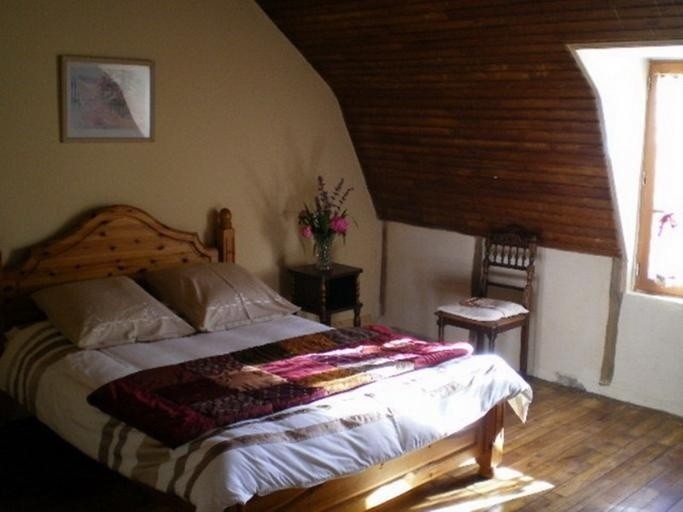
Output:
[
  {"x1": 30, "y1": 275, "x2": 196, "y2": 350},
  {"x1": 141, "y1": 263, "x2": 301, "y2": 330}
]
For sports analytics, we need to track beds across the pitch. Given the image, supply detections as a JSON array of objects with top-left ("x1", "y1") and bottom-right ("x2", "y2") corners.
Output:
[{"x1": 0, "y1": 204, "x2": 532, "y2": 512}]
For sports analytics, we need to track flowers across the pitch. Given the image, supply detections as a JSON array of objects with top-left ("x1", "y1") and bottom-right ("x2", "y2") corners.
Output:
[{"x1": 300, "y1": 176, "x2": 354, "y2": 239}]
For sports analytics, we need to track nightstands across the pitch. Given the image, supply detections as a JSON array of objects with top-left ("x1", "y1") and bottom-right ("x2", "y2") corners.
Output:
[{"x1": 288, "y1": 262, "x2": 363, "y2": 329}]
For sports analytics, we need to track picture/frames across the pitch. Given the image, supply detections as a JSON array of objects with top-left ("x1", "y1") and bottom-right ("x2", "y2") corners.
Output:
[{"x1": 58, "y1": 56, "x2": 156, "y2": 142}]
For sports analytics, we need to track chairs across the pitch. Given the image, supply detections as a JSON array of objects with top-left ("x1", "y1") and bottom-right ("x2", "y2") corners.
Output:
[{"x1": 433, "y1": 226, "x2": 537, "y2": 381}]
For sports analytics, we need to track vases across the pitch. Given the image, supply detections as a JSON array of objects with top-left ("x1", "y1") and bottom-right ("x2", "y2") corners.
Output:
[{"x1": 317, "y1": 239, "x2": 332, "y2": 272}]
